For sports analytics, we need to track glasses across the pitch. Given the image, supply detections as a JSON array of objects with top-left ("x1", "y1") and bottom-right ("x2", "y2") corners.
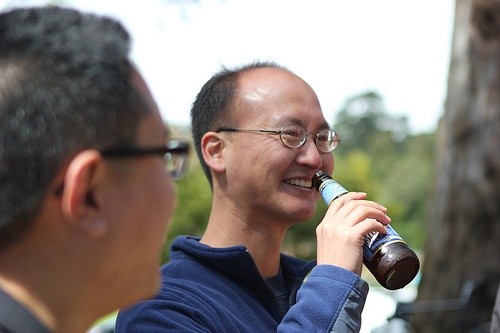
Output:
[
  {"x1": 52, "y1": 139, "x2": 193, "y2": 197},
  {"x1": 216, "y1": 124, "x2": 341, "y2": 156}
]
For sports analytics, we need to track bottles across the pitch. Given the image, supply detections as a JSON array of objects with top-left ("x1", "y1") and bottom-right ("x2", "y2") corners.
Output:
[{"x1": 311, "y1": 169, "x2": 420, "y2": 291}]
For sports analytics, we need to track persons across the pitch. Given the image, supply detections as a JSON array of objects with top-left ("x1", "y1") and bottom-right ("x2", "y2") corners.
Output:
[
  {"x1": 0, "y1": 2, "x2": 177, "y2": 330},
  {"x1": 119, "y1": 62, "x2": 394, "y2": 332}
]
